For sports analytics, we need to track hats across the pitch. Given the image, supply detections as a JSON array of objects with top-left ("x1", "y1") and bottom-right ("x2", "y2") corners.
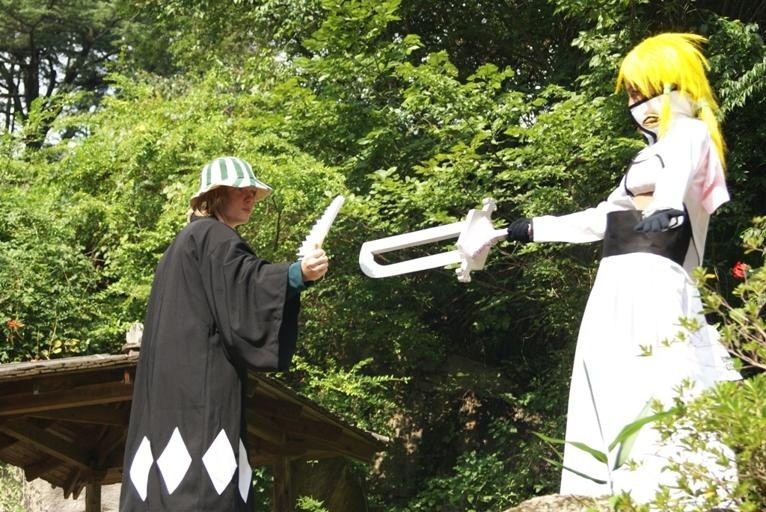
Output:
[{"x1": 190, "y1": 156, "x2": 273, "y2": 211}]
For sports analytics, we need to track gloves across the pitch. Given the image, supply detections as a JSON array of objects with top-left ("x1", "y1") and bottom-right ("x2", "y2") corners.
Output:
[
  {"x1": 633, "y1": 209, "x2": 686, "y2": 232},
  {"x1": 507, "y1": 218, "x2": 534, "y2": 246}
]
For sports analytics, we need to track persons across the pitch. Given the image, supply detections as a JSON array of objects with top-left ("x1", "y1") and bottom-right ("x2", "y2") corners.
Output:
[
  {"x1": 506, "y1": 31, "x2": 750, "y2": 510},
  {"x1": 117, "y1": 157, "x2": 329, "y2": 512}
]
